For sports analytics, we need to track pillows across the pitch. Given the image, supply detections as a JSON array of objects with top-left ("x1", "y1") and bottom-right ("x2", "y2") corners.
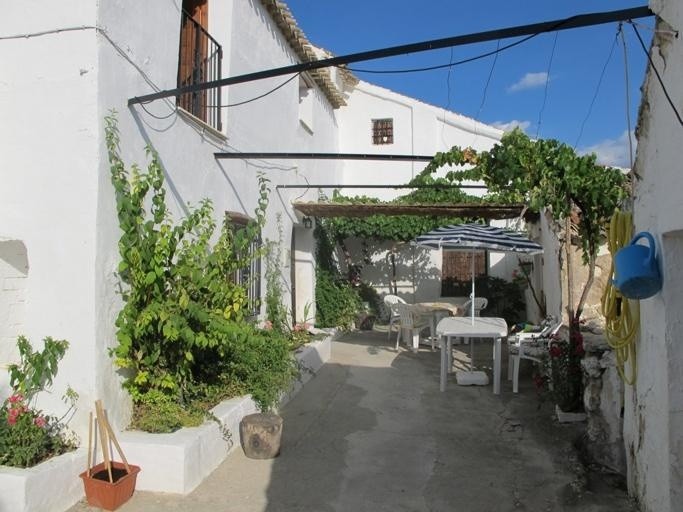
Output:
[
  {"x1": 508, "y1": 321, "x2": 533, "y2": 335},
  {"x1": 524, "y1": 324, "x2": 544, "y2": 333}
]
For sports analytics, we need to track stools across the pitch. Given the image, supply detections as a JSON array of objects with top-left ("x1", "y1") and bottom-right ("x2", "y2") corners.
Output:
[{"x1": 239, "y1": 411, "x2": 283, "y2": 459}]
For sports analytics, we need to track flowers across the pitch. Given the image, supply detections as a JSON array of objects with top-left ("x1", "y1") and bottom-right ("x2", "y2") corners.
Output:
[
  {"x1": 0, "y1": 394, "x2": 44, "y2": 462},
  {"x1": 532, "y1": 318, "x2": 587, "y2": 412}
]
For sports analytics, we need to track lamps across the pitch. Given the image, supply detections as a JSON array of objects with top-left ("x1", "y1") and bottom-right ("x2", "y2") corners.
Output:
[{"x1": 305, "y1": 215, "x2": 312, "y2": 229}]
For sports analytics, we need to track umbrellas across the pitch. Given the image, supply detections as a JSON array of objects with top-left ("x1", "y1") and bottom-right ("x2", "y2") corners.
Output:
[{"x1": 398, "y1": 221, "x2": 545, "y2": 375}]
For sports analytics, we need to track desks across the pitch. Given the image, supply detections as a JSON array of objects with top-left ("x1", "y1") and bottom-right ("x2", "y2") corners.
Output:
[{"x1": 436, "y1": 316, "x2": 508, "y2": 394}]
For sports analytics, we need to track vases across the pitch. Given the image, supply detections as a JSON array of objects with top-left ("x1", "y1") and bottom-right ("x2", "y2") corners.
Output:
[
  {"x1": 556, "y1": 404, "x2": 586, "y2": 423},
  {"x1": 79, "y1": 462, "x2": 140, "y2": 512}
]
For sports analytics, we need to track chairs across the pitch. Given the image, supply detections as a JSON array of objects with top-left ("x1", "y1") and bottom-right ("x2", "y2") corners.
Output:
[
  {"x1": 508, "y1": 313, "x2": 564, "y2": 394},
  {"x1": 383, "y1": 294, "x2": 488, "y2": 353}
]
